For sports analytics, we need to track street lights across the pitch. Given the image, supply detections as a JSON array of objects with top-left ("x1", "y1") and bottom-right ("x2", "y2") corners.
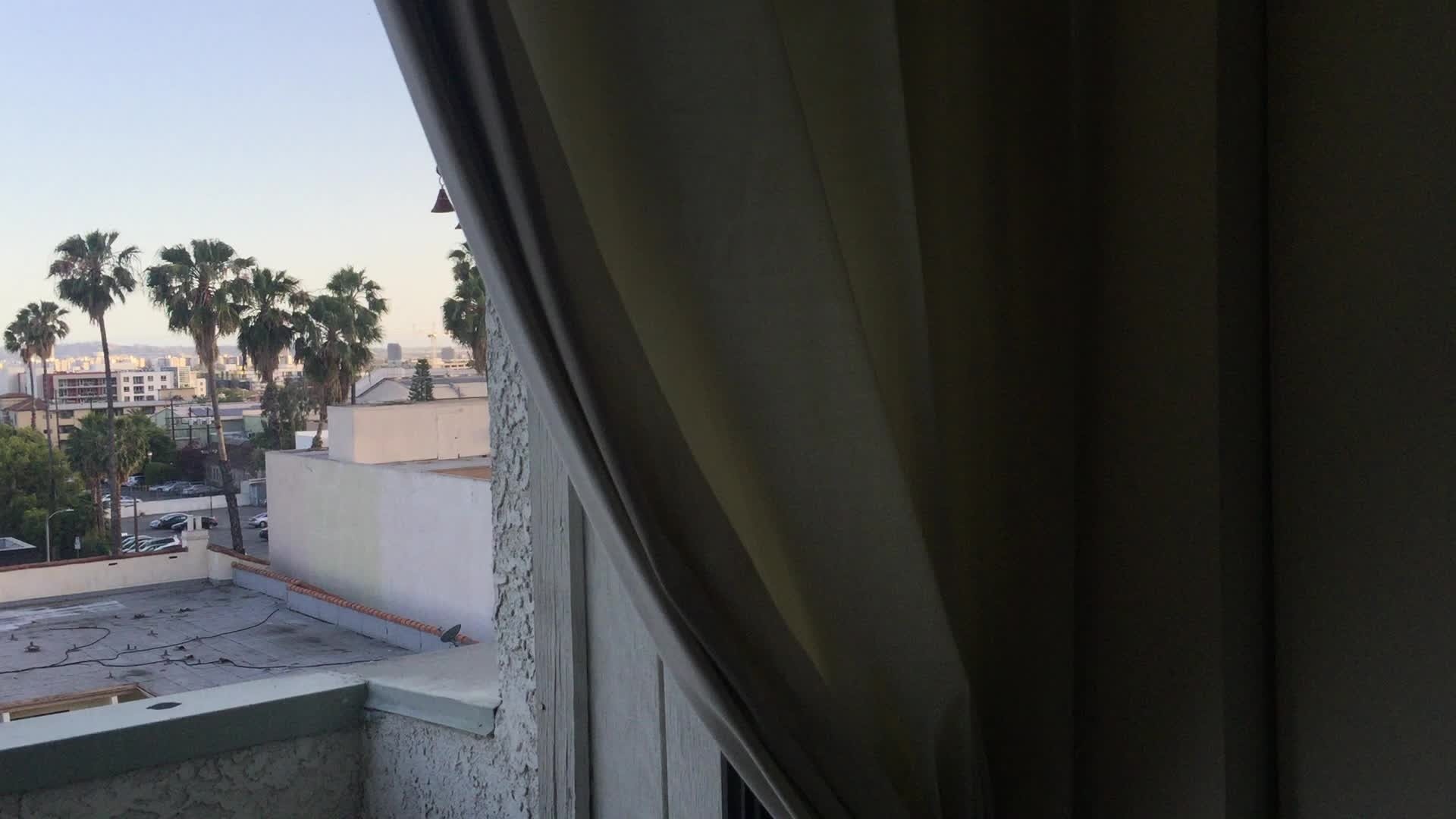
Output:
[{"x1": 46, "y1": 509, "x2": 74, "y2": 562}]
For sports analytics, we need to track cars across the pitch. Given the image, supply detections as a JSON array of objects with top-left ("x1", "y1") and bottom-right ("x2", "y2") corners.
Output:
[
  {"x1": 149, "y1": 481, "x2": 173, "y2": 492},
  {"x1": 259, "y1": 527, "x2": 268, "y2": 540},
  {"x1": 182, "y1": 484, "x2": 204, "y2": 497},
  {"x1": 248, "y1": 513, "x2": 268, "y2": 528},
  {"x1": 150, "y1": 513, "x2": 194, "y2": 529},
  {"x1": 162, "y1": 482, "x2": 192, "y2": 493},
  {"x1": 171, "y1": 516, "x2": 218, "y2": 533},
  {"x1": 122, "y1": 535, "x2": 182, "y2": 553},
  {"x1": 126, "y1": 476, "x2": 142, "y2": 486},
  {"x1": 194, "y1": 486, "x2": 224, "y2": 497},
  {"x1": 103, "y1": 494, "x2": 142, "y2": 505}
]
